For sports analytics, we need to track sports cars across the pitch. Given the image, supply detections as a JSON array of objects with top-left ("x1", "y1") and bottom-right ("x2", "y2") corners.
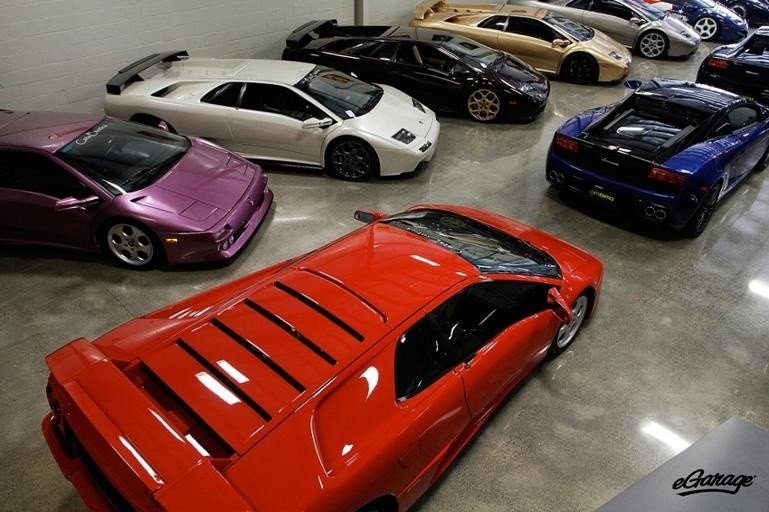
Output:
[{"x1": 41, "y1": 204, "x2": 605, "y2": 512}]
